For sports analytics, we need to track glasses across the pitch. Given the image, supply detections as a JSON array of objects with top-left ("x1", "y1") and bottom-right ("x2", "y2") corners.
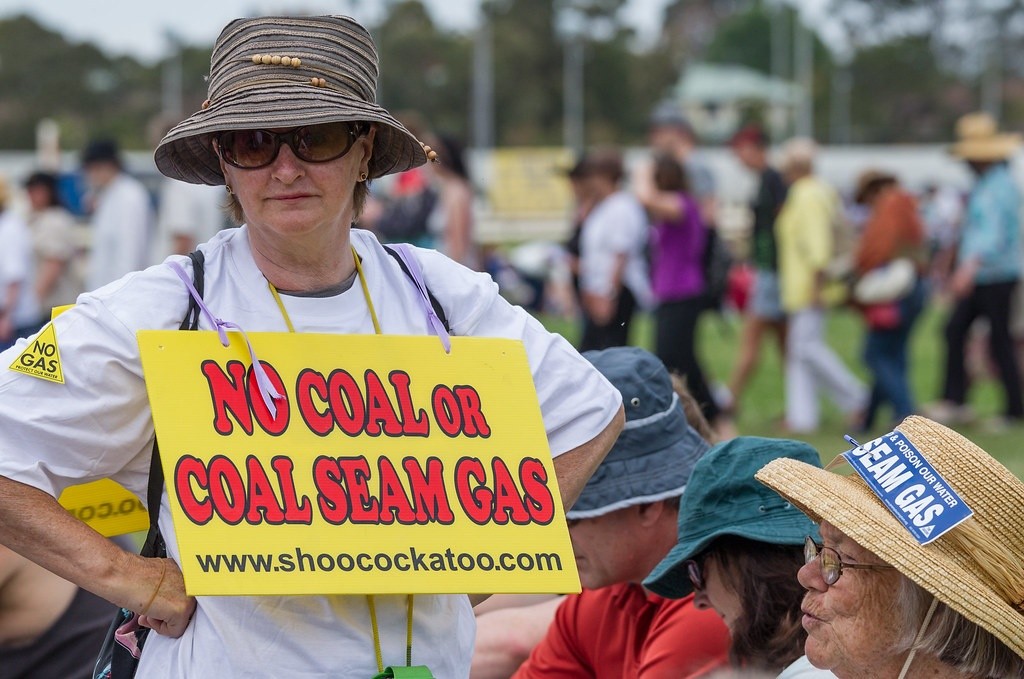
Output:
[
  {"x1": 215, "y1": 122, "x2": 371, "y2": 170},
  {"x1": 803, "y1": 535, "x2": 897, "y2": 585},
  {"x1": 683, "y1": 548, "x2": 715, "y2": 592}
]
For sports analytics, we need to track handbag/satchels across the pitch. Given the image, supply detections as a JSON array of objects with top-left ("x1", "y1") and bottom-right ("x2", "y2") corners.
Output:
[{"x1": 852, "y1": 256, "x2": 916, "y2": 306}]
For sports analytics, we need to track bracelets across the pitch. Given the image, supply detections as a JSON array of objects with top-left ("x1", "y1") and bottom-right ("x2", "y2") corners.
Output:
[{"x1": 141, "y1": 556, "x2": 166, "y2": 616}]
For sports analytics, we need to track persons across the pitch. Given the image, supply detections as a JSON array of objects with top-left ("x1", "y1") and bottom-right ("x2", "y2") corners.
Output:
[
  {"x1": 0, "y1": 16, "x2": 627, "y2": 679},
  {"x1": 0, "y1": 106, "x2": 1024, "y2": 434},
  {"x1": 468, "y1": 346, "x2": 1024, "y2": 679}
]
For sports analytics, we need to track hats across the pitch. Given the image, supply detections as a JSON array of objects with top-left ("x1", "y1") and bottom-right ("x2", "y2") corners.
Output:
[
  {"x1": 557, "y1": 144, "x2": 626, "y2": 185},
  {"x1": 943, "y1": 111, "x2": 1020, "y2": 160},
  {"x1": 642, "y1": 435, "x2": 824, "y2": 600},
  {"x1": 754, "y1": 415, "x2": 1024, "y2": 661},
  {"x1": 563, "y1": 346, "x2": 713, "y2": 520},
  {"x1": 852, "y1": 167, "x2": 895, "y2": 206},
  {"x1": 153, "y1": 15, "x2": 441, "y2": 186},
  {"x1": 80, "y1": 138, "x2": 117, "y2": 164}
]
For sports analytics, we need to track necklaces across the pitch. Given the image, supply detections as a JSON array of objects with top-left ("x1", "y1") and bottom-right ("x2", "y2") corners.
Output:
[{"x1": 267, "y1": 248, "x2": 435, "y2": 679}]
